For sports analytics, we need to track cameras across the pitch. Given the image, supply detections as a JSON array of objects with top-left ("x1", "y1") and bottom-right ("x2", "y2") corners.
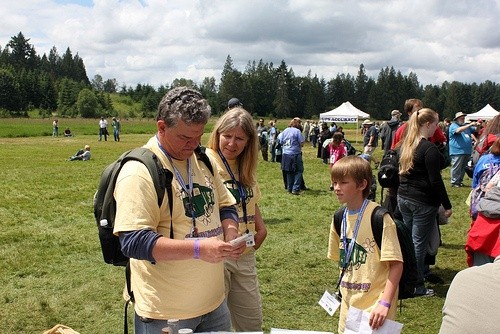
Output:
[{"x1": 475, "y1": 122, "x2": 481, "y2": 126}]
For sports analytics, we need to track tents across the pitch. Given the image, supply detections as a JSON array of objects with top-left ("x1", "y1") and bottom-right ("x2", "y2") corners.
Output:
[
  {"x1": 320, "y1": 101, "x2": 370, "y2": 144},
  {"x1": 465, "y1": 104, "x2": 500, "y2": 123}
]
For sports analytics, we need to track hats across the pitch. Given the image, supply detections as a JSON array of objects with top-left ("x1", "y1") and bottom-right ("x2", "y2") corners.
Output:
[
  {"x1": 453, "y1": 111, "x2": 466, "y2": 120},
  {"x1": 361, "y1": 119, "x2": 373, "y2": 125},
  {"x1": 228, "y1": 98, "x2": 243, "y2": 107}
]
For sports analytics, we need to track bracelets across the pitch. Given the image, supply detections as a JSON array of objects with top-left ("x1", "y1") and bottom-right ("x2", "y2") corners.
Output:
[
  {"x1": 194, "y1": 239, "x2": 200, "y2": 259},
  {"x1": 378, "y1": 300, "x2": 391, "y2": 309}
]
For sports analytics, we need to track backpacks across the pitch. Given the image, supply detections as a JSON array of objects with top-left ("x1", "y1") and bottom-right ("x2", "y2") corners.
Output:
[
  {"x1": 333, "y1": 205, "x2": 416, "y2": 300},
  {"x1": 471, "y1": 165, "x2": 500, "y2": 216},
  {"x1": 93, "y1": 145, "x2": 214, "y2": 266}
]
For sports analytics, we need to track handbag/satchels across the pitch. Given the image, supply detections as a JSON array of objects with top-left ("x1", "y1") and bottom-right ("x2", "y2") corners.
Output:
[
  {"x1": 378, "y1": 150, "x2": 400, "y2": 189},
  {"x1": 476, "y1": 187, "x2": 500, "y2": 219}
]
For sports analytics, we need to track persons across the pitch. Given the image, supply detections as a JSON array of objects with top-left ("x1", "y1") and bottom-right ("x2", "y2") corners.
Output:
[
  {"x1": 69, "y1": 145, "x2": 91, "y2": 161},
  {"x1": 98, "y1": 116, "x2": 109, "y2": 141},
  {"x1": 114, "y1": 86, "x2": 246, "y2": 334},
  {"x1": 206, "y1": 108, "x2": 268, "y2": 332},
  {"x1": 64, "y1": 127, "x2": 72, "y2": 137},
  {"x1": 327, "y1": 155, "x2": 404, "y2": 334},
  {"x1": 228, "y1": 98, "x2": 500, "y2": 334},
  {"x1": 111, "y1": 117, "x2": 121, "y2": 142},
  {"x1": 52, "y1": 118, "x2": 59, "y2": 137}
]
